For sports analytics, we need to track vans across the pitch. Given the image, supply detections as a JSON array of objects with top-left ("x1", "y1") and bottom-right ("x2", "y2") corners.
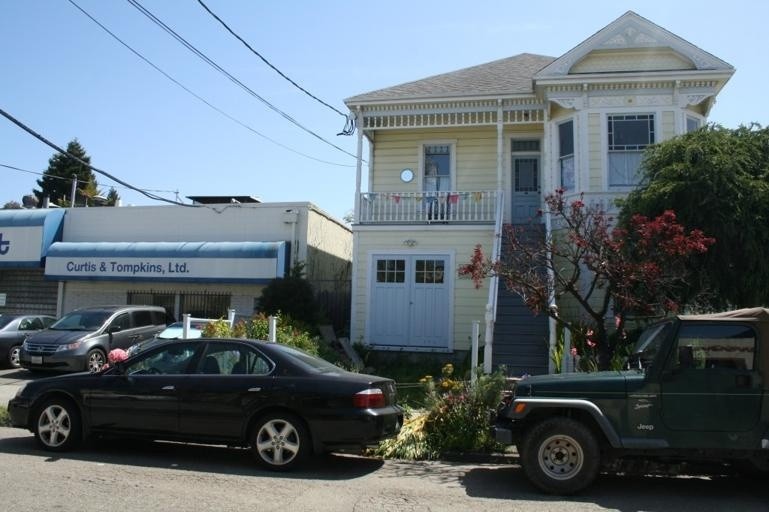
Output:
[{"x1": 16, "y1": 306, "x2": 166, "y2": 378}]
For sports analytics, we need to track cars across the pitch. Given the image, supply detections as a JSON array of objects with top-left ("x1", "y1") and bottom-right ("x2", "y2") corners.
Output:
[
  {"x1": 5, "y1": 336, "x2": 405, "y2": 469},
  {"x1": 159, "y1": 319, "x2": 209, "y2": 337},
  {"x1": 0, "y1": 313, "x2": 61, "y2": 368}
]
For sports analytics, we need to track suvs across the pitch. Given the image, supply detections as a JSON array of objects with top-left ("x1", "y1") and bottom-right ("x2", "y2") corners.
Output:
[{"x1": 495, "y1": 305, "x2": 769, "y2": 493}]
